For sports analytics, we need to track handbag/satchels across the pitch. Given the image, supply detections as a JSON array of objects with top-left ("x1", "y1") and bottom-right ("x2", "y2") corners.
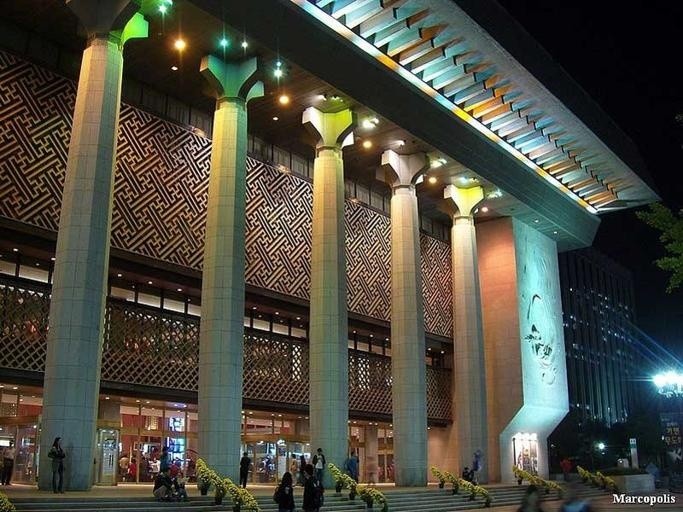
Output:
[{"x1": 48, "y1": 452, "x2": 55, "y2": 458}]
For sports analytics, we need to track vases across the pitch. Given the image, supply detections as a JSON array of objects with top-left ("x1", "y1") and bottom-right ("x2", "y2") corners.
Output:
[{"x1": 485, "y1": 502, "x2": 491, "y2": 507}]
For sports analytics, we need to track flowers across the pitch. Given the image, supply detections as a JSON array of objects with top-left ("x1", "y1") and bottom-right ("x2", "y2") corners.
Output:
[
  {"x1": 327, "y1": 462, "x2": 387, "y2": 512},
  {"x1": 430, "y1": 467, "x2": 491, "y2": 502},
  {"x1": 195, "y1": 459, "x2": 261, "y2": 512},
  {"x1": 512, "y1": 465, "x2": 618, "y2": 499}
]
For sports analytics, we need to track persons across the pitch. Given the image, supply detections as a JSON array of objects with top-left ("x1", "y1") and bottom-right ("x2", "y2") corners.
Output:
[
  {"x1": 344, "y1": 451, "x2": 358, "y2": 485},
  {"x1": 667, "y1": 447, "x2": 682, "y2": 464},
  {"x1": 463, "y1": 450, "x2": 484, "y2": 487},
  {"x1": 290, "y1": 453, "x2": 299, "y2": 486},
  {"x1": 299, "y1": 455, "x2": 305, "y2": 487},
  {"x1": 302, "y1": 464, "x2": 324, "y2": 512},
  {"x1": 2, "y1": 442, "x2": 16, "y2": 485},
  {"x1": 273, "y1": 471, "x2": 296, "y2": 512},
  {"x1": 310, "y1": 447, "x2": 326, "y2": 486},
  {"x1": 239, "y1": 452, "x2": 251, "y2": 490},
  {"x1": 48, "y1": 437, "x2": 66, "y2": 493},
  {"x1": 118, "y1": 446, "x2": 183, "y2": 502},
  {"x1": 560, "y1": 457, "x2": 572, "y2": 482}
]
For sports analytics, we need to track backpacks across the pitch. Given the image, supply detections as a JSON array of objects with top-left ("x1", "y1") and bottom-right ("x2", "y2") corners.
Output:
[
  {"x1": 313, "y1": 480, "x2": 323, "y2": 507},
  {"x1": 315, "y1": 454, "x2": 323, "y2": 469}
]
